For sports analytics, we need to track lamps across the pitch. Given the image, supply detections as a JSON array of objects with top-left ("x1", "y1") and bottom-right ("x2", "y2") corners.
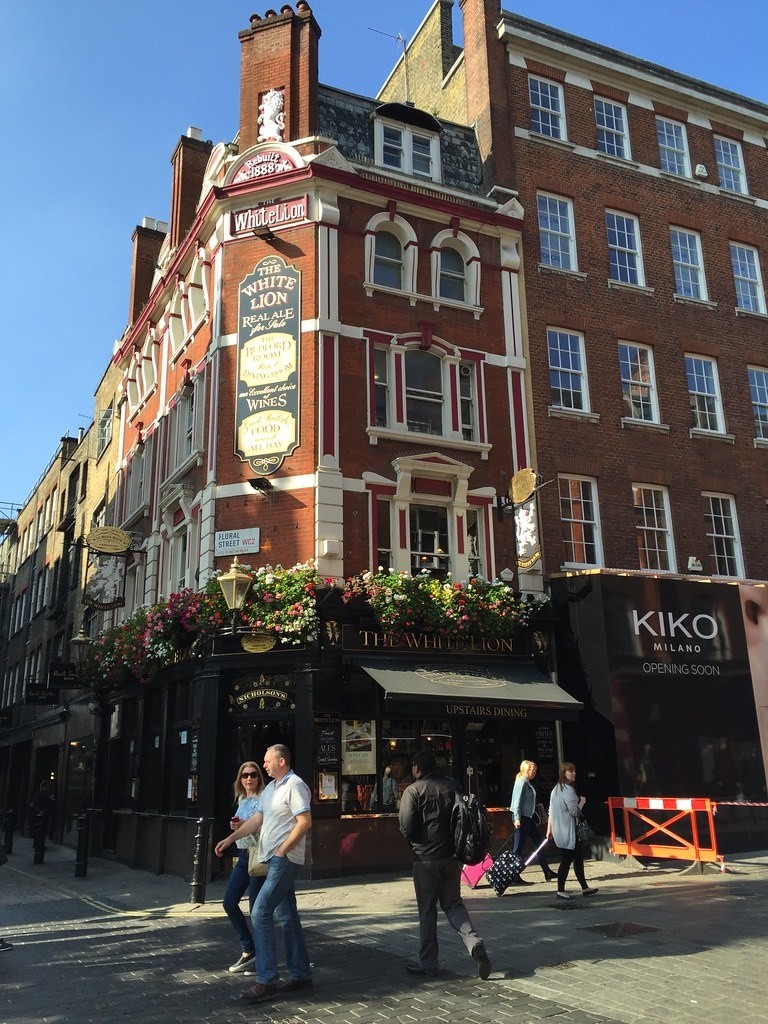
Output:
[
  {"x1": 210, "y1": 555, "x2": 269, "y2": 639},
  {"x1": 247, "y1": 477, "x2": 273, "y2": 496}
]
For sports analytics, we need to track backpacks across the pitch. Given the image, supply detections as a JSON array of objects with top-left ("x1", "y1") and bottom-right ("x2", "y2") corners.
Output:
[{"x1": 441, "y1": 775, "x2": 494, "y2": 866}]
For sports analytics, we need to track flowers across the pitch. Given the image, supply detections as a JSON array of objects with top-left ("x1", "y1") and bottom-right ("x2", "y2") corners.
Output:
[{"x1": 80, "y1": 558, "x2": 551, "y2": 682}]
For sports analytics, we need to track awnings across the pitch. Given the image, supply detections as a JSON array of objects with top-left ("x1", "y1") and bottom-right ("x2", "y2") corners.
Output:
[{"x1": 356, "y1": 659, "x2": 584, "y2": 712}]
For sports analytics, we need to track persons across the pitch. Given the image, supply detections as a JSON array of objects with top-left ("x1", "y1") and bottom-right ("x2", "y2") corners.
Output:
[
  {"x1": 29, "y1": 780, "x2": 56, "y2": 851},
  {"x1": 397, "y1": 749, "x2": 494, "y2": 980},
  {"x1": 213, "y1": 743, "x2": 312, "y2": 1003},
  {"x1": 543, "y1": 761, "x2": 599, "y2": 900},
  {"x1": 368, "y1": 766, "x2": 399, "y2": 813},
  {"x1": 221, "y1": 760, "x2": 268, "y2": 974},
  {"x1": 511, "y1": 760, "x2": 558, "y2": 886}
]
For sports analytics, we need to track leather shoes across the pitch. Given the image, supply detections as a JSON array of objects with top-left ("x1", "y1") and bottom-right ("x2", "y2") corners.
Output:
[
  {"x1": 405, "y1": 964, "x2": 439, "y2": 976},
  {"x1": 238, "y1": 977, "x2": 313, "y2": 1002},
  {"x1": 546, "y1": 873, "x2": 558, "y2": 882},
  {"x1": 582, "y1": 887, "x2": 599, "y2": 896},
  {"x1": 555, "y1": 892, "x2": 574, "y2": 900},
  {"x1": 472, "y1": 943, "x2": 490, "y2": 983}
]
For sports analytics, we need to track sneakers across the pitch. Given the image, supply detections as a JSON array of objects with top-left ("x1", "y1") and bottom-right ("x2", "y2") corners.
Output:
[{"x1": 229, "y1": 951, "x2": 259, "y2": 976}]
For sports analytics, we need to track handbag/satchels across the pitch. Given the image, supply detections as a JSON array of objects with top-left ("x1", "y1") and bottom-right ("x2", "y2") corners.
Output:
[
  {"x1": 574, "y1": 806, "x2": 595, "y2": 847},
  {"x1": 247, "y1": 845, "x2": 269, "y2": 877}
]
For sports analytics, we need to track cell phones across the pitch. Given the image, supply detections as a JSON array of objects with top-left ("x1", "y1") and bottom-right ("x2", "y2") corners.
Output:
[{"x1": 231, "y1": 816, "x2": 239, "y2": 822}]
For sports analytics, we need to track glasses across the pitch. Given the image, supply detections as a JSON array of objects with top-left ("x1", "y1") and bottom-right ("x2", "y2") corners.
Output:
[{"x1": 240, "y1": 772, "x2": 259, "y2": 779}]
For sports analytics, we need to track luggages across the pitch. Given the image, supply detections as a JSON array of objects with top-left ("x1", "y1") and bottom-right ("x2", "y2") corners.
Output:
[
  {"x1": 462, "y1": 824, "x2": 521, "y2": 889},
  {"x1": 485, "y1": 835, "x2": 553, "y2": 897}
]
